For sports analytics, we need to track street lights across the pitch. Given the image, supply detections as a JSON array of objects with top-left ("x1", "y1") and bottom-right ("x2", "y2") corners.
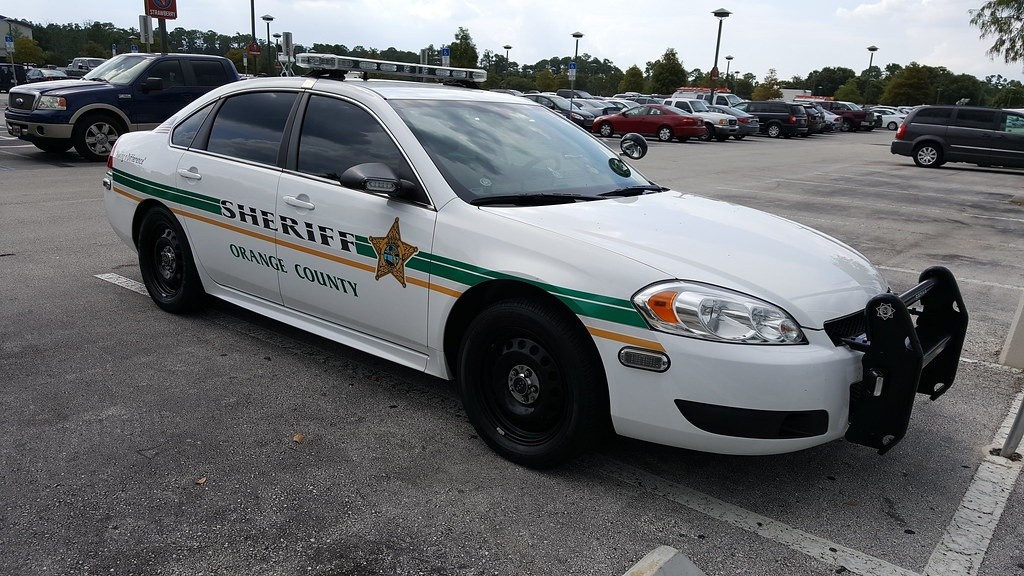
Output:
[
  {"x1": 712, "y1": 7, "x2": 732, "y2": 68},
  {"x1": 724, "y1": 55, "x2": 742, "y2": 94},
  {"x1": 571, "y1": 30, "x2": 584, "y2": 62},
  {"x1": 271, "y1": 33, "x2": 282, "y2": 78},
  {"x1": 503, "y1": 45, "x2": 512, "y2": 91},
  {"x1": 865, "y1": 45, "x2": 880, "y2": 104},
  {"x1": 261, "y1": 14, "x2": 275, "y2": 77}
]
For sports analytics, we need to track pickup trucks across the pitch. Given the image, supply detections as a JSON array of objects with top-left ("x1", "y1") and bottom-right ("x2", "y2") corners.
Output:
[{"x1": 3, "y1": 52, "x2": 242, "y2": 163}]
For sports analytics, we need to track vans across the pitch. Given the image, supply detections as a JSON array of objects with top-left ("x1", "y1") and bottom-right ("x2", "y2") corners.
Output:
[{"x1": 891, "y1": 107, "x2": 1024, "y2": 168}]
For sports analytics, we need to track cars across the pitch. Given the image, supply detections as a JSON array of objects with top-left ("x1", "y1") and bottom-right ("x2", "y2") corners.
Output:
[
  {"x1": 590, "y1": 103, "x2": 708, "y2": 143},
  {"x1": 102, "y1": 52, "x2": 971, "y2": 475},
  {"x1": 0, "y1": 57, "x2": 109, "y2": 95},
  {"x1": 490, "y1": 84, "x2": 935, "y2": 140}
]
